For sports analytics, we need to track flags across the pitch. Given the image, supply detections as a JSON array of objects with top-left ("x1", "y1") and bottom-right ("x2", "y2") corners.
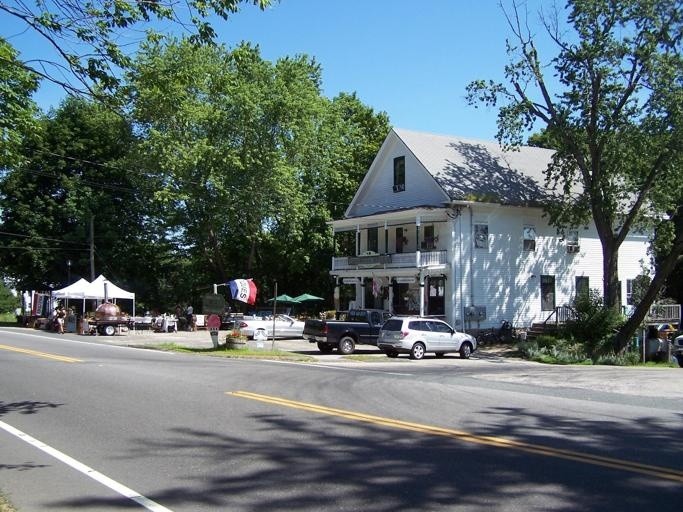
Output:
[
  {"x1": 223, "y1": 279, "x2": 258, "y2": 305},
  {"x1": 372, "y1": 273, "x2": 384, "y2": 299}
]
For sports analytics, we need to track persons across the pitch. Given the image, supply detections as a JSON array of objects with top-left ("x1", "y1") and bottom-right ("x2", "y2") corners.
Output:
[
  {"x1": 186, "y1": 303, "x2": 193, "y2": 329},
  {"x1": 57, "y1": 303, "x2": 66, "y2": 334}
]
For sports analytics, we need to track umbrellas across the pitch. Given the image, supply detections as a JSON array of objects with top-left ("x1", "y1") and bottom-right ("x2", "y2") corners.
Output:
[
  {"x1": 268, "y1": 294, "x2": 293, "y2": 303},
  {"x1": 293, "y1": 293, "x2": 326, "y2": 303}
]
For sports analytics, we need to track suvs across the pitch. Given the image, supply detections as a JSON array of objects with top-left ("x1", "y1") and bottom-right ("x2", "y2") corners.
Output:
[{"x1": 376, "y1": 316, "x2": 477, "y2": 358}]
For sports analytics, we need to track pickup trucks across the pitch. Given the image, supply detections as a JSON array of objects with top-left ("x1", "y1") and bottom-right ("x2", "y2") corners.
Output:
[{"x1": 302, "y1": 307, "x2": 395, "y2": 354}]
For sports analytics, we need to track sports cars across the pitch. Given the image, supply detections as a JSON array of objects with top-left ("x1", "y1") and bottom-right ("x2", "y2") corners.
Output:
[{"x1": 236, "y1": 314, "x2": 305, "y2": 341}]
